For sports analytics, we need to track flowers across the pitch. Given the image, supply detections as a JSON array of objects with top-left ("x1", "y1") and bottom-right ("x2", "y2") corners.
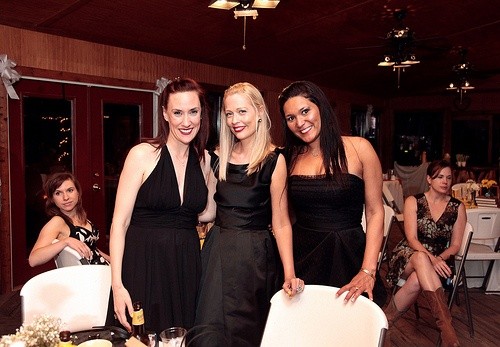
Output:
[
  {"x1": 480, "y1": 179, "x2": 497, "y2": 189},
  {"x1": 467, "y1": 183, "x2": 481, "y2": 192}
]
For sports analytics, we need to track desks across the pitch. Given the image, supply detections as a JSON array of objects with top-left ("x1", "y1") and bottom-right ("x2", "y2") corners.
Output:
[{"x1": 396, "y1": 206, "x2": 500, "y2": 295}]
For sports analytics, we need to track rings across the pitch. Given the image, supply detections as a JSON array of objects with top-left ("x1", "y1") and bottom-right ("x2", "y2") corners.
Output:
[
  {"x1": 114, "y1": 314, "x2": 117, "y2": 319},
  {"x1": 298, "y1": 286, "x2": 301, "y2": 290},
  {"x1": 354, "y1": 286, "x2": 358, "y2": 291}
]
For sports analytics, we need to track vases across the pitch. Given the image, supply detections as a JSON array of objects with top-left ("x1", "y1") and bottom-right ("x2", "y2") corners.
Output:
[
  {"x1": 471, "y1": 192, "x2": 478, "y2": 208},
  {"x1": 485, "y1": 189, "x2": 494, "y2": 198}
]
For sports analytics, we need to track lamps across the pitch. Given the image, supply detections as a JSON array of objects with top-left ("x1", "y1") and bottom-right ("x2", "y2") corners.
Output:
[
  {"x1": 378, "y1": 10, "x2": 421, "y2": 88},
  {"x1": 446, "y1": 48, "x2": 476, "y2": 104},
  {"x1": 208, "y1": 0, "x2": 280, "y2": 50}
]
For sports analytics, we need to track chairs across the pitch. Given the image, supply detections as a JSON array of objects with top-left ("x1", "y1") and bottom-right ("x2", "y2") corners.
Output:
[
  {"x1": 20, "y1": 265, "x2": 111, "y2": 335},
  {"x1": 415, "y1": 223, "x2": 474, "y2": 347},
  {"x1": 52, "y1": 239, "x2": 91, "y2": 269},
  {"x1": 259, "y1": 285, "x2": 389, "y2": 347},
  {"x1": 382, "y1": 185, "x2": 407, "y2": 250},
  {"x1": 361, "y1": 205, "x2": 394, "y2": 274},
  {"x1": 453, "y1": 208, "x2": 500, "y2": 305}
]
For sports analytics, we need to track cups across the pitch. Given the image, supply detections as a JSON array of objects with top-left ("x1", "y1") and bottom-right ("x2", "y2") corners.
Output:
[
  {"x1": 78, "y1": 339, "x2": 112, "y2": 347},
  {"x1": 160, "y1": 327, "x2": 188, "y2": 347},
  {"x1": 196, "y1": 225, "x2": 208, "y2": 239}
]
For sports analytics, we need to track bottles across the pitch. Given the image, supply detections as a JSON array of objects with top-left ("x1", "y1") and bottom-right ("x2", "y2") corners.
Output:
[
  {"x1": 470, "y1": 191, "x2": 478, "y2": 209},
  {"x1": 389, "y1": 170, "x2": 396, "y2": 180},
  {"x1": 59, "y1": 330, "x2": 72, "y2": 347},
  {"x1": 128, "y1": 301, "x2": 151, "y2": 347}
]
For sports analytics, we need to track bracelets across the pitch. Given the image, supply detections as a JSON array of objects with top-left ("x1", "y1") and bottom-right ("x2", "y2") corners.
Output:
[
  {"x1": 439, "y1": 256, "x2": 443, "y2": 260},
  {"x1": 362, "y1": 268, "x2": 375, "y2": 281}
]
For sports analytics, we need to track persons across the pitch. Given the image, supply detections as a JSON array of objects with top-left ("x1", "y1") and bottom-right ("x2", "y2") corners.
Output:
[
  {"x1": 384, "y1": 160, "x2": 466, "y2": 347},
  {"x1": 186, "y1": 81, "x2": 304, "y2": 347},
  {"x1": 278, "y1": 81, "x2": 386, "y2": 302},
  {"x1": 29, "y1": 173, "x2": 110, "y2": 268},
  {"x1": 106, "y1": 78, "x2": 212, "y2": 342}
]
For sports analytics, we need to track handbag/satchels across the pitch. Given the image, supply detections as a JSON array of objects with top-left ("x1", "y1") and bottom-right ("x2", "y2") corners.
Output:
[{"x1": 69, "y1": 325, "x2": 131, "y2": 346}]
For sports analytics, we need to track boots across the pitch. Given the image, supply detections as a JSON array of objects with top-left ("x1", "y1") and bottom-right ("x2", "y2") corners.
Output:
[
  {"x1": 383, "y1": 295, "x2": 414, "y2": 347},
  {"x1": 423, "y1": 286, "x2": 462, "y2": 347}
]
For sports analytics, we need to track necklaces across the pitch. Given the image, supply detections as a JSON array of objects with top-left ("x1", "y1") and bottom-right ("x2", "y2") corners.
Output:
[
  {"x1": 310, "y1": 152, "x2": 318, "y2": 157},
  {"x1": 173, "y1": 151, "x2": 187, "y2": 166}
]
